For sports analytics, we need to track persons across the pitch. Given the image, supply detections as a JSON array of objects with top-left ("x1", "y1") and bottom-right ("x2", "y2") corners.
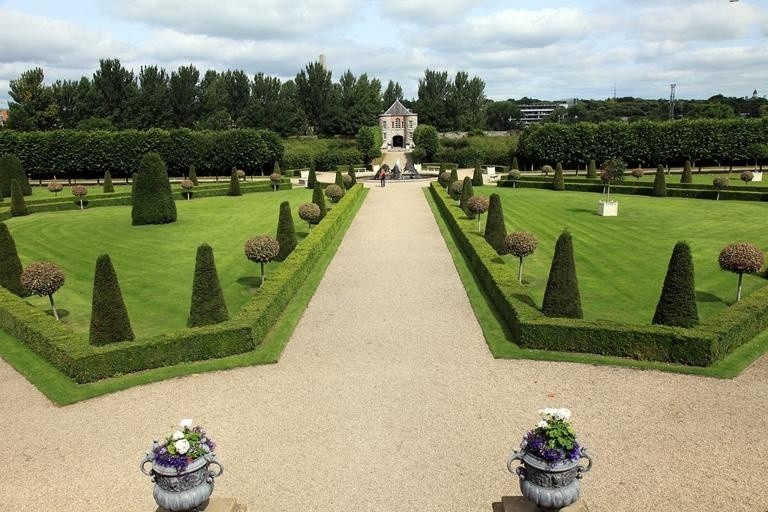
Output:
[{"x1": 378, "y1": 168, "x2": 386, "y2": 188}]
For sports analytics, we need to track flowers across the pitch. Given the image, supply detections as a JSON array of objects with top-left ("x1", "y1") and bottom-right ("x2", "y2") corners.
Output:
[
  {"x1": 524, "y1": 406, "x2": 584, "y2": 466},
  {"x1": 153, "y1": 415, "x2": 216, "y2": 468}
]
